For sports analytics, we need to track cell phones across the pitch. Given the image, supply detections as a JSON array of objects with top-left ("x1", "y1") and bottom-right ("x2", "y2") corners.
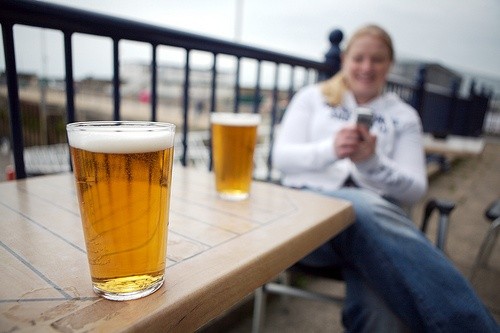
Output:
[{"x1": 355, "y1": 108, "x2": 373, "y2": 141}]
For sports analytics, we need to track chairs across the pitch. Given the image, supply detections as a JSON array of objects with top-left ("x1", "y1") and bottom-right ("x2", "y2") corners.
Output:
[
  {"x1": 251, "y1": 197, "x2": 454, "y2": 333},
  {"x1": 475, "y1": 199, "x2": 500, "y2": 269}
]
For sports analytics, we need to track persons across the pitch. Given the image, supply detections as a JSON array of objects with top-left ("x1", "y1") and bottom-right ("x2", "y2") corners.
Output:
[{"x1": 271, "y1": 24, "x2": 500, "y2": 333}]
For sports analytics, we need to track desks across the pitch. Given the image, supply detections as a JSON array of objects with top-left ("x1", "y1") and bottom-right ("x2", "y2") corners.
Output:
[
  {"x1": 0, "y1": 163, "x2": 358, "y2": 333},
  {"x1": 423, "y1": 135, "x2": 485, "y2": 182}
]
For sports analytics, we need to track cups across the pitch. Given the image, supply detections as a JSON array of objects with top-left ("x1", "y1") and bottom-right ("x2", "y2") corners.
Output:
[
  {"x1": 209, "y1": 112, "x2": 261, "y2": 201},
  {"x1": 66, "y1": 120, "x2": 176, "y2": 302}
]
